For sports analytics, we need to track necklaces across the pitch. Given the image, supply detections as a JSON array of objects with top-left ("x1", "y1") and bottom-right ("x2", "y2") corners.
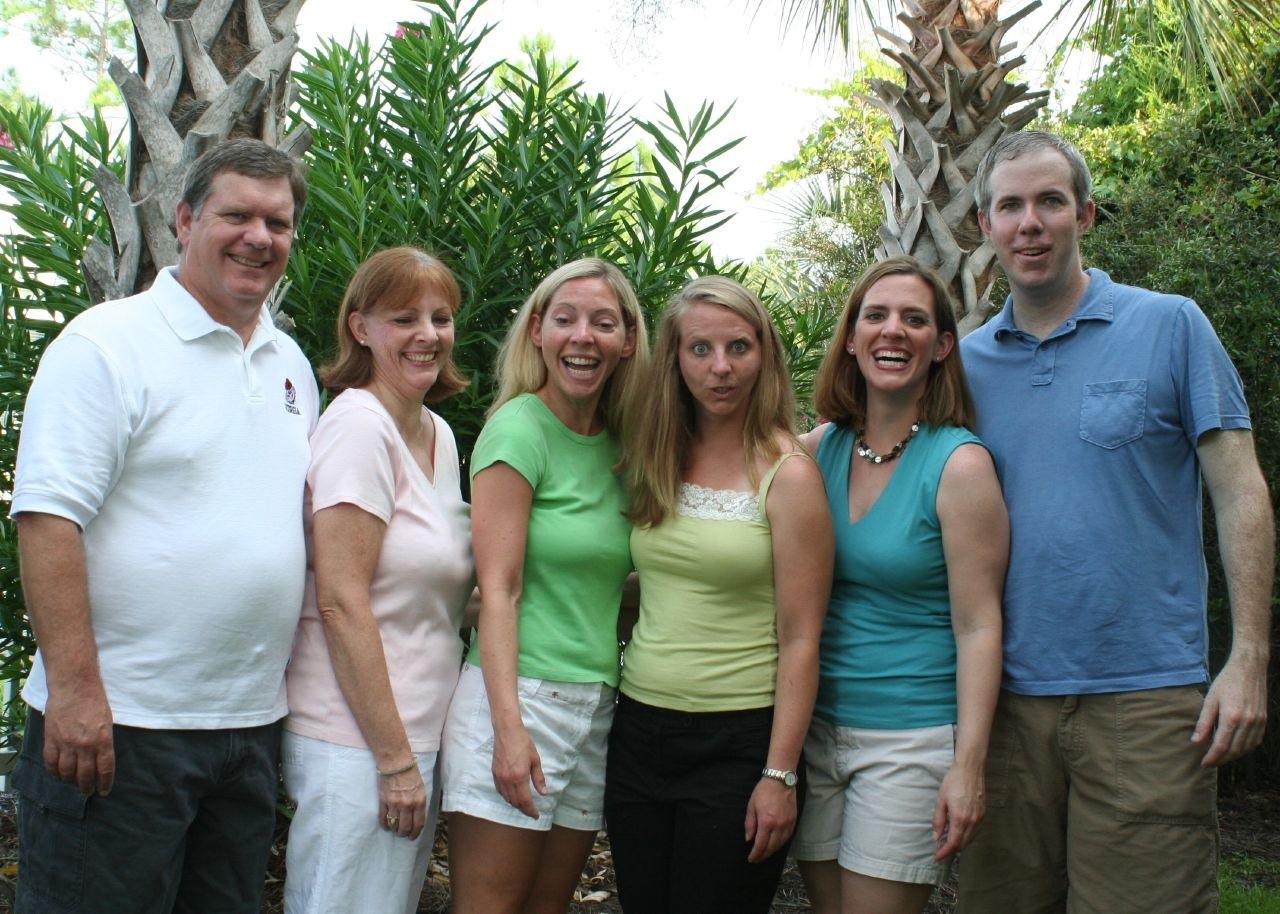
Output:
[{"x1": 856, "y1": 418, "x2": 923, "y2": 464}]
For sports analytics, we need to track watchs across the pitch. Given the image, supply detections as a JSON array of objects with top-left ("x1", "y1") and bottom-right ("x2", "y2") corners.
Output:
[{"x1": 763, "y1": 768, "x2": 799, "y2": 788}]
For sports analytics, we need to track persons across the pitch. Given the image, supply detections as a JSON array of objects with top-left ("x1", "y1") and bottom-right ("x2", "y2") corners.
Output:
[
  {"x1": 796, "y1": 256, "x2": 1013, "y2": 914},
  {"x1": 13, "y1": 137, "x2": 321, "y2": 914},
  {"x1": 955, "y1": 130, "x2": 1275, "y2": 914},
  {"x1": 281, "y1": 246, "x2": 835, "y2": 914}
]
